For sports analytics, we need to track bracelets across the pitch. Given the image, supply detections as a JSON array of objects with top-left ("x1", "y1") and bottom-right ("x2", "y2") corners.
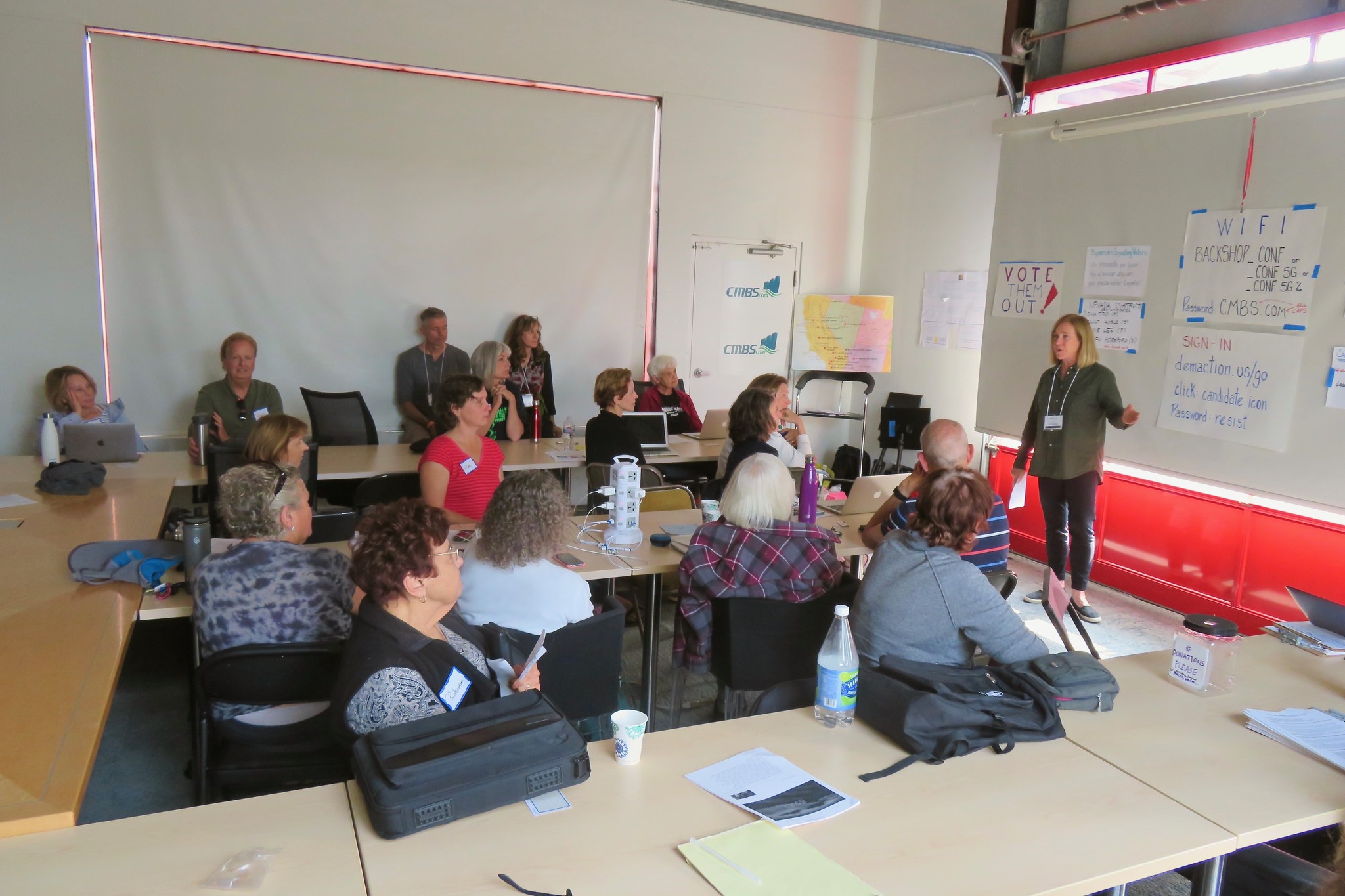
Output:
[
  {"x1": 893, "y1": 486, "x2": 910, "y2": 501},
  {"x1": 427, "y1": 422, "x2": 435, "y2": 429}
]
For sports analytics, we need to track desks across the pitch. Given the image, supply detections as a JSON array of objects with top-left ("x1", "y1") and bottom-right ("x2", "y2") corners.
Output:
[
  {"x1": 541, "y1": 432, "x2": 726, "y2": 506},
  {"x1": 318, "y1": 434, "x2": 564, "y2": 520},
  {"x1": 565, "y1": 503, "x2": 877, "y2": 737},
  {"x1": 0, "y1": 477, "x2": 176, "y2": 847},
  {"x1": 0, "y1": 451, "x2": 207, "y2": 503},
  {"x1": 346, "y1": 698, "x2": 1235, "y2": 896},
  {"x1": 139, "y1": 519, "x2": 632, "y2": 810},
  {"x1": 0, "y1": 781, "x2": 367, "y2": 896},
  {"x1": 1058, "y1": 633, "x2": 1345, "y2": 896}
]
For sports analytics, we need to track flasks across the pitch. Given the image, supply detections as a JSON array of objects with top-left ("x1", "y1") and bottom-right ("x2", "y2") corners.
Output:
[
  {"x1": 530, "y1": 400, "x2": 542, "y2": 443},
  {"x1": 181, "y1": 514, "x2": 211, "y2": 594},
  {"x1": 41, "y1": 412, "x2": 60, "y2": 467},
  {"x1": 192, "y1": 413, "x2": 210, "y2": 466}
]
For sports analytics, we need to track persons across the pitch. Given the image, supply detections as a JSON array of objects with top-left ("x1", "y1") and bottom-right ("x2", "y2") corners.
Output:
[
  {"x1": 186, "y1": 308, "x2": 845, "y2": 748},
  {"x1": 34, "y1": 364, "x2": 150, "y2": 452},
  {"x1": 1011, "y1": 315, "x2": 1140, "y2": 622},
  {"x1": 862, "y1": 419, "x2": 1010, "y2": 572},
  {"x1": 846, "y1": 465, "x2": 1050, "y2": 669}
]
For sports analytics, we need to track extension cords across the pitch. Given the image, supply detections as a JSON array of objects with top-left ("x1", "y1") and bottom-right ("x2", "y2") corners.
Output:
[{"x1": 603, "y1": 455, "x2": 644, "y2": 545}]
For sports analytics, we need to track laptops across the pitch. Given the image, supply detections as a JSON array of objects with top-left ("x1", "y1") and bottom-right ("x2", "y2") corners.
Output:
[
  {"x1": 621, "y1": 412, "x2": 678, "y2": 456},
  {"x1": 681, "y1": 408, "x2": 731, "y2": 440},
  {"x1": 1286, "y1": 586, "x2": 1345, "y2": 637},
  {"x1": 817, "y1": 473, "x2": 912, "y2": 515},
  {"x1": 63, "y1": 422, "x2": 141, "y2": 463}
]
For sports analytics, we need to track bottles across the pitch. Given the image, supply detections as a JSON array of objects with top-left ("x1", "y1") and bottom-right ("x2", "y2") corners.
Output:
[
  {"x1": 815, "y1": 604, "x2": 859, "y2": 729},
  {"x1": 1167, "y1": 613, "x2": 1244, "y2": 698},
  {"x1": 798, "y1": 454, "x2": 819, "y2": 524},
  {"x1": 563, "y1": 416, "x2": 575, "y2": 456},
  {"x1": 778, "y1": 425, "x2": 782, "y2": 431}
]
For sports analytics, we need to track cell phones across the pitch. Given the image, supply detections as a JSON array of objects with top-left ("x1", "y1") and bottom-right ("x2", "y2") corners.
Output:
[{"x1": 553, "y1": 553, "x2": 585, "y2": 568}]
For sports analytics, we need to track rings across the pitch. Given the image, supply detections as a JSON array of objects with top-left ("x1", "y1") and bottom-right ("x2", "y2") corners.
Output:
[
  {"x1": 527, "y1": 684, "x2": 530, "y2": 690},
  {"x1": 791, "y1": 440, "x2": 794, "y2": 443}
]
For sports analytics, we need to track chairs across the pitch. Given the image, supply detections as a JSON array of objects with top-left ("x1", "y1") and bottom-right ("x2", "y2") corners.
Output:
[{"x1": 187, "y1": 370, "x2": 1017, "y2": 807}]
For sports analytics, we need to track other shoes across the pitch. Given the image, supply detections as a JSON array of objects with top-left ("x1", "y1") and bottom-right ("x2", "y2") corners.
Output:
[
  {"x1": 1023, "y1": 587, "x2": 1067, "y2": 603},
  {"x1": 1069, "y1": 597, "x2": 1101, "y2": 623},
  {"x1": 603, "y1": 592, "x2": 647, "y2": 627}
]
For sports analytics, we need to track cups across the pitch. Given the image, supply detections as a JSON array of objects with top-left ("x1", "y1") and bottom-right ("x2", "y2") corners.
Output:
[
  {"x1": 788, "y1": 478, "x2": 796, "y2": 522},
  {"x1": 610, "y1": 709, "x2": 648, "y2": 766},
  {"x1": 818, "y1": 487, "x2": 829, "y2": 501},
  {"x1": 700, "y1": 499, "x2": 719, "y2": 524},
  {"x1": 706, "y1": 510, "x2": 722, "y2": 522}
]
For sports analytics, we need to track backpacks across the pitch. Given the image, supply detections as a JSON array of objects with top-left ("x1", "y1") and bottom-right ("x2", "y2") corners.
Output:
[{"x1": 865, "y1": 654, "x2": 1066, "y2": 783}]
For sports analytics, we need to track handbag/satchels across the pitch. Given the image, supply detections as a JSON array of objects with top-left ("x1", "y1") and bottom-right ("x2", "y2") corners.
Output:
[
  {"x1": 353, "y1": 687, "x2": 592, "y2": 840},
  {"x1": 1005, "y1": 650, "x2": 1120, "y2": 712}
]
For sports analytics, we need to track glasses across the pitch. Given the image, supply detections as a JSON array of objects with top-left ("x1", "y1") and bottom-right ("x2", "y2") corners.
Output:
[
  {"x1": 252, "y1": 458, "x2": 286, "y2": 505},
  {"x1": 236, "y1": 399, "x2": 247, "y2": 423},
  {"x1": 427, "y1": 545, "x2": 459, "y2": 562}
]
